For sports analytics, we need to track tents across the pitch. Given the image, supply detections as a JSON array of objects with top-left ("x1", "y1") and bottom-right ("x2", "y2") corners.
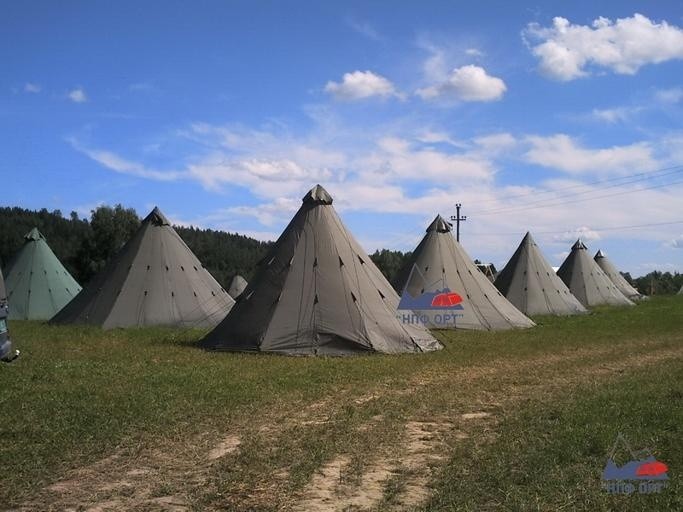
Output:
[
  {"x1": 487, "y1": 230, "x2": 586, "y2": 318},
  {"x1": 391, "y1": 213, "x2": 536, "y2": 337},
  {"x1": 226, "y1": 275, "x2": 248, "y2": 299},
  {"x1": 2, "y1": 225, "x2": 86, "y2": 321},
  {"x1": 555, "y1": 236, "x2": 637, "y2": 310},
  {"x1": 44, "y1": 204, "x2": 236, "y2": 331},
  {"x1": 592, "y1": 248, "x2": 648, "y2": 300},
  {"x1": 192, "y1": 183, "x2": 445, "y2": 357}
]
